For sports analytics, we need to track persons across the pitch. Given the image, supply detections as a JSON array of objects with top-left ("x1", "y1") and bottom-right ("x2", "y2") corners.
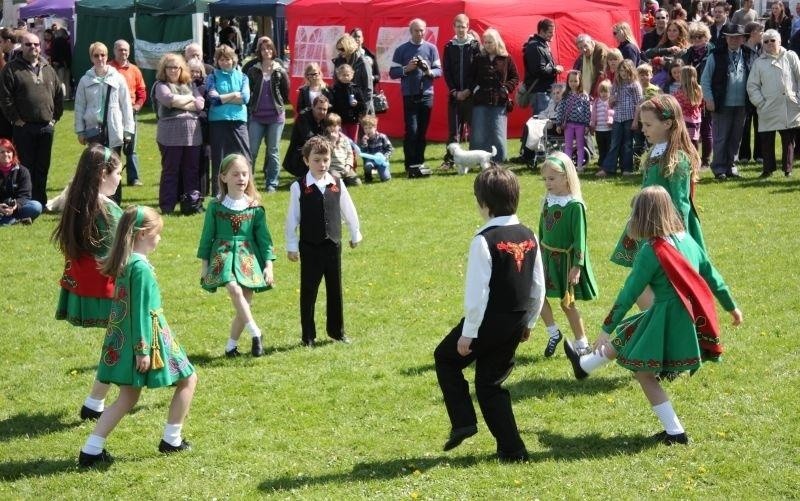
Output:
[
  {"x1": 561, "y1": 186, "x2": 745, "y2": 448},
  {"x1": 285, "y1": 135, "x2": 362, "y2": 347},
  {"x1": 75, "y1": 39, "x2": 134, "y2": 208},
  {"x1": 46, "y1": 145, "x2": 122, "y2": 422},
  {"x1": 100, "y1": 40, "x2": 147, "y2": 190},
  {"x1": 608, "y1": 94, "x2": 711, "y2": 312},
  {"x1": 537, "y1": 151, "x2": 596, "y2": 359},
  {"x1": 197, "y1": 155, "x2": 276, "y2": 361},
  {"x1": 434, "y1": 165, "x2": 546, "y2": 464},
  {"x1": 1, "y1": 12, "x2": 73, "y2": 228},
  {"x1": 389, "y1": 17, "x2": 444, "y2": 178},
  {"x1": 75, "y1": 207, "x2": 199, "y2": 471}
]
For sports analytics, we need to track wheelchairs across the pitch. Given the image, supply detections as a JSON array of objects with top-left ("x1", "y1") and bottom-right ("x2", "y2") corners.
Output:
[{"x1": 520, "y1": 114, "x2": 594, "y2": 169}]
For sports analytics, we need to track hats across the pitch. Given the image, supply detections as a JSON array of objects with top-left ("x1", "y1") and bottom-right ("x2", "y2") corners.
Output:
[{"x1": 720, "y1": 23, "x2": 750, "y2": 37}]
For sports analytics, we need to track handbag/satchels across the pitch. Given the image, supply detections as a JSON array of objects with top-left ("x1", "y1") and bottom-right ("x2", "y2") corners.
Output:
[
  {"x1": 507, "y1": 97, "x2": 513, "y2": 112},
  {"x1": 83, "y1": 126, "x2": 108, "y2": 143},
  {"x1": 515, "y1": 83, "x2": 529, "y2": 107},
  {"x1": 373, "y1": 91, "x2": 389, "y2": 113}
]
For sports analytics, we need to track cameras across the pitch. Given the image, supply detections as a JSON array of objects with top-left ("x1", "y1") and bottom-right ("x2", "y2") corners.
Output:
[
  {"x1": 410, "y1": 55, "x2": 429, "y2": 72},
  {"x1": 4, "y1": 197, "x2": 16, "y2": 206}
]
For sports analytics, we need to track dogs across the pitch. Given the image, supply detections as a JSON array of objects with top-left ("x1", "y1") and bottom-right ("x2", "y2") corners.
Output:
[
  {"x1": 46, "y1": 179, "x2": 73, "y2": 213},
  {"x1": 446, "y1": 142, "x2": 498, "y2": 176}
]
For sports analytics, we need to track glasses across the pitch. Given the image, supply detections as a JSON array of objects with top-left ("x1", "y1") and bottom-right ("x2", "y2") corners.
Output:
[
  {"x1": 92, "y1": 46, "x2": 345, "y2": 81},
  {"x1": 765, "y1": 38, "x2": 779, "y2": 44},
  {"x1": 24, "y1": 42, "x2": 41, "y2": 47}
]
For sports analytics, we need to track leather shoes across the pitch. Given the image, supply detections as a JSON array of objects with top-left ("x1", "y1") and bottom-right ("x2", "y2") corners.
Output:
[
  {"x1": 303, "y1": 336, "x2": 317, "y2": 348},
  {"x1": 487, "y1": 451, "x2": 530, "y2": 463},
  {"x1": 251, "y1": 331, "x2": 265, "y2": 356},
  {"x1": 80, "y1": 404, "x2": 104, "y2": 421},
  {"x1": 78, "y1": 448, "x2": 114, "y2": 468},
  {"x1": 328, "y1": 330, "x2": 349, "y2": 343},
  {"x1": 442, "y1": 424, "x2": 479, "y2": 451},
  {"x1": 225, "y1": 347, "x2": 242, "y2": 356},
  {"x1": 544, "y1": 329, "x2": 593, "y2": 382},
  {"x1": 654, "y1": 430, "x2": 689, "y2": 445},
  {"x1": 158, "y1": 437, "x2": 190, "y2": 454}
]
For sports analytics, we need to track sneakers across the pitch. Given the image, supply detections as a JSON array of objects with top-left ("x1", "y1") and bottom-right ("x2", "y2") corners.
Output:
[
  {"x1": 13, "y1": 218, "x2": 33, "y2": 226},
  {"x1": 577, "y1": 162, "x2": 634, "y2": 179},
  {"x1": 406, "y1": 152, "x2": 533, "y2": 180},
  {"x1": 700, "y1": 157, "x2": 793, "y2": 180},
  {"x1": 265, "y1": 184, "x2": 279, "y2": 193},
  {"x1": 127, "y1": 180, "x2": 143, "y2": 186}
]
